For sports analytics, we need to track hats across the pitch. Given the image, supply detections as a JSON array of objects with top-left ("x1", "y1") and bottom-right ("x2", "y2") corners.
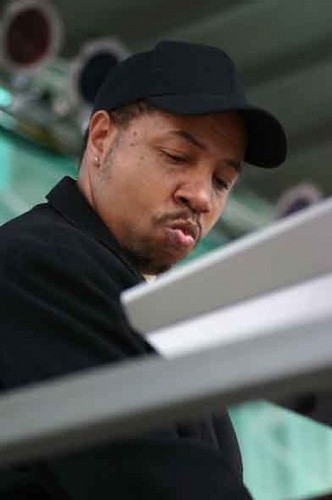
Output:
[{"x1": 91, "y1": 39, "x2": 286, "y2": 169}]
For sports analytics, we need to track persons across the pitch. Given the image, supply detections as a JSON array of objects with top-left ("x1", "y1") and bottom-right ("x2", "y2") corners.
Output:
[{"x1": 0, "y1": 41, "x2": 292, "y2": 500}]
